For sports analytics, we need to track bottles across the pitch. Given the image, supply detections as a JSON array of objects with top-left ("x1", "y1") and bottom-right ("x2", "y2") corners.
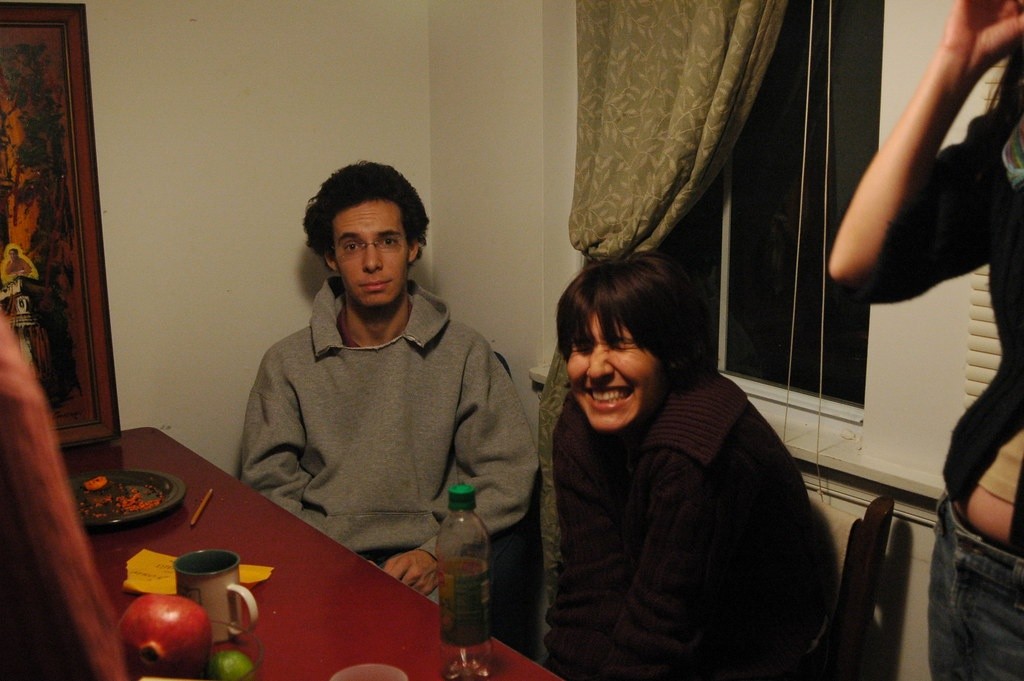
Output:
[{"x1": 435, "y1": 483, "x2": 493, "y2": 679}]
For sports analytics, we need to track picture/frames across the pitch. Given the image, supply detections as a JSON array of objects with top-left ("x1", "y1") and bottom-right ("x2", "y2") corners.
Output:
[{"x1": 0, "y1": 2, "x2": 122, "y2": 450}]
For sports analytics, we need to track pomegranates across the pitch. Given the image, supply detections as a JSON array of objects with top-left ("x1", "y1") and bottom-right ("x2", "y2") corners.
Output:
[{"x1": 119, "y1": 594, "x2": 212, "y2": 679}]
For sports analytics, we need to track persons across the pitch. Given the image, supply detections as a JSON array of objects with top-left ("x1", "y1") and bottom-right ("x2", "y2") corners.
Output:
[
  {"x1": 829, "y1": 0, "x2": 1024, "y2": 681},
  {"x1": 536, "y1": 249, "x2": 820, "y2": 681},
  {"x1": 236, "y1": 161, "x2": 540, "y2": 609}
]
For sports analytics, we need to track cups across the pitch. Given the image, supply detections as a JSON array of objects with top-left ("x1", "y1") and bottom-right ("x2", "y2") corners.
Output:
[
  {"x1": 173, "y1": 550, "x2": 257, "y2": 641},
  {"x1": 330, "y1": 664, "x2": 408, "y2": 681}
]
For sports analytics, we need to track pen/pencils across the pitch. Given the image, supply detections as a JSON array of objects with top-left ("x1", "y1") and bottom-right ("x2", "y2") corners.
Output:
[{"x1": 190, "y1": 488, "x2": 214, "y2": 525}]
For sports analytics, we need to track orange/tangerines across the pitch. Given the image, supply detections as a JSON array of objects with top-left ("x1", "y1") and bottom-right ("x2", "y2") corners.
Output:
[{"x1": 209, "y1": 651, "x2": 254, "y2": 681}]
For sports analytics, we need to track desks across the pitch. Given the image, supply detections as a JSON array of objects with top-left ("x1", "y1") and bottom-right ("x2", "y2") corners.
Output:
[{"x1": 58, "y1": 426, "x2": 566, "y2": 681}]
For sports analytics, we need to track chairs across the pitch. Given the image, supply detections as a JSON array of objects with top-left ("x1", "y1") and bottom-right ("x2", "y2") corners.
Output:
[{"x1": 828, "y1": 495, "x2": 894, "y2": 681}]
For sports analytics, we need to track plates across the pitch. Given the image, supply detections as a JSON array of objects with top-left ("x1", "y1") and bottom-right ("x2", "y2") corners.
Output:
[{"x1": 69, "y1": 467, "x2": 187, "y2": 535}]
